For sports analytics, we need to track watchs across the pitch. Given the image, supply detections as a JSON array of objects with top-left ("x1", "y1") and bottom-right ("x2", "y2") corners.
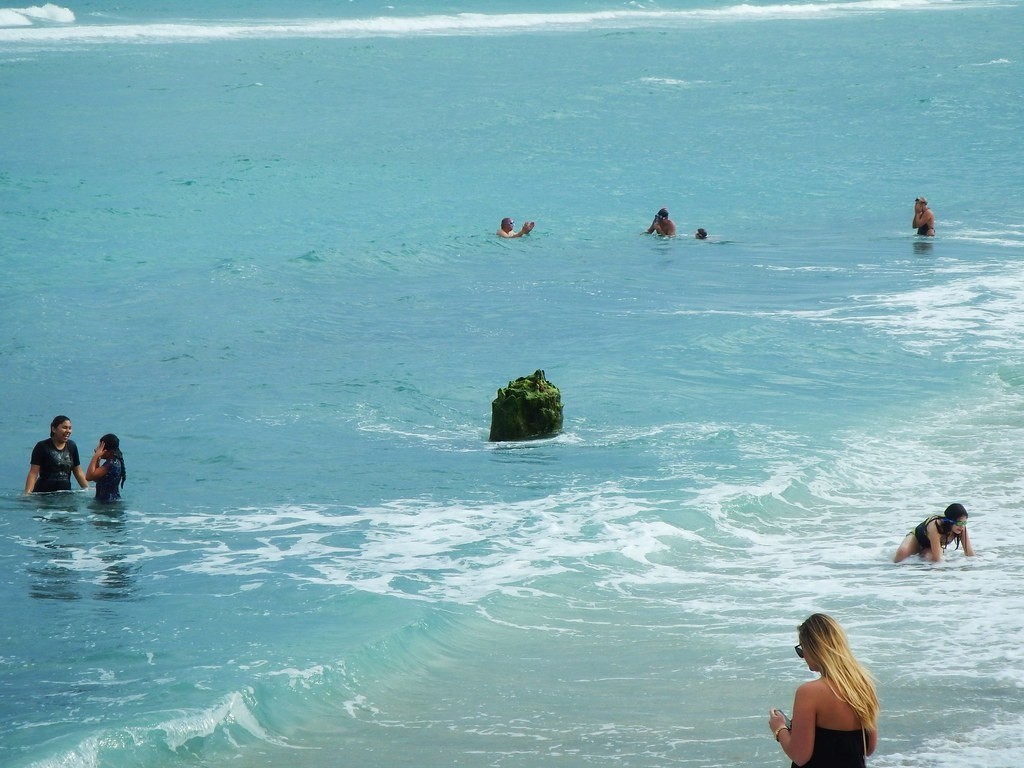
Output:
[{"x1": 774, "y1": 725, "x2": 789, "y2": 743}]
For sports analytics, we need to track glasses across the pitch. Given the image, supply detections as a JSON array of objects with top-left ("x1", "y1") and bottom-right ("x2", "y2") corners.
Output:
[
  {"x1": 795, "y1": 641, "x2": 808, "y2": 658},
  {"x1": 957, "y1": 521, "x2": 967, "y2": 526}
]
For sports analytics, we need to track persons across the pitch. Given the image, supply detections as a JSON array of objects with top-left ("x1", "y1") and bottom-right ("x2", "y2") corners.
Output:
[
  {"x1": 768, "y1": 613, "x2": 880, "y2": 768},
  {"x1": 696, "y1": 228, "x2": 708, "y2": 240},
  {"x1": 85, "y1": 434, "x2": 127, "y2": 500},
  {"x1": 912, "y1": 197, "x2": 935, "y2": 236},
  {"x1": 26, "y1": 414, "x2": 88, "y2": 494},
  {"x1": 647, "y1": 208, "x2": 676, "y2": 237},
  {"x1": 496, "y1": 218, "x2": 534, "y2": 238},
  {"x1": 893, "y1": 503, "x2": 975, "y2": 563}
]
